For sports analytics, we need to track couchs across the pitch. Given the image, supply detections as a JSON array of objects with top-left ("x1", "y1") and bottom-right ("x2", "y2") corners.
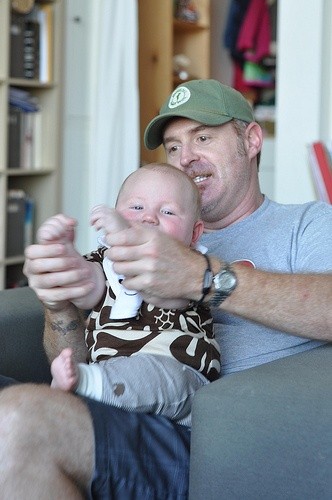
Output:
[{"x1": 0, "y1": 287, "x2": 332, "y2": 500}]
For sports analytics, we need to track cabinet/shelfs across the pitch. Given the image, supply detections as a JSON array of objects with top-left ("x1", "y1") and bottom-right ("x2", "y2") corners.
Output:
[
  {"x1": 139, "y1": 0, "x2": 212, "y2": 168},
  {"x1": 0, "y1": 0, "x2": 64, "y2": 291}
]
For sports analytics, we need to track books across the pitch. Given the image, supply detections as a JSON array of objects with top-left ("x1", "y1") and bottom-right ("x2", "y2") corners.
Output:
[
  {"x1": 8, "y1": 85, "x2": 43, "y2": 169},
  {"x1": 9, "y1": 7, "x2": 48, "y2": 80},
  {"x1": 5, "y1": 188, "x2": 34, "y2": 258}
]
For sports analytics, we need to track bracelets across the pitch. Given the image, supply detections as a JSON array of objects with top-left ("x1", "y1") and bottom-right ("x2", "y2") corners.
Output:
[{"x1": 193, "y1": 253, "x2": 215, "y2": 304}]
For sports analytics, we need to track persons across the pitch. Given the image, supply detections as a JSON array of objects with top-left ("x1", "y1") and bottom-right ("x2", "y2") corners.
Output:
[
  {"x1": 36, "y1": 162, "x2": 221, "y2": 428},
  {"x1": 0, "y1": 78, "x2": 332, "y2": 500}
]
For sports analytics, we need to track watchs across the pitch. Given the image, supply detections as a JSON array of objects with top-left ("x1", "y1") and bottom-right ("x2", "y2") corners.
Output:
[{"x1": 206, "y1": 259, "x2": 238, "y2": 307}]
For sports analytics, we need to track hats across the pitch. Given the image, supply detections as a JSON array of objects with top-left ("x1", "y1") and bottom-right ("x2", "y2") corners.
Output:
[{"x1": 144, "y1": 78, "x2": 256, "y2": 151}]
